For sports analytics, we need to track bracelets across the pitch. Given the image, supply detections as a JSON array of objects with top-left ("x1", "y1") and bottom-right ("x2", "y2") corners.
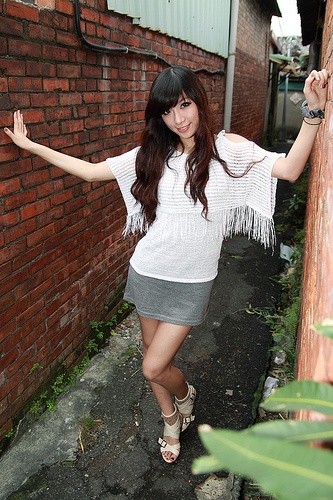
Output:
[
  {"x1": 302, "y1": 117, "x2": 322, "y2": 125},
  {"x1": 301, "y1": 100, "x2": 325, "y2": 119}
]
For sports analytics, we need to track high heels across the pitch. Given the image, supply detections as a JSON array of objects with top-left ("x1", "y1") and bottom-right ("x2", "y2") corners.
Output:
[{"x1": 158, "y1": 382, "x2": 199, "y2": 463}]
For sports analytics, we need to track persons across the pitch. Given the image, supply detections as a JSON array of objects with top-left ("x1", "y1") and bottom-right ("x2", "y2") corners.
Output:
[{"x1": 4, "y1": 65, "x2": 329, "y2": 463}]
